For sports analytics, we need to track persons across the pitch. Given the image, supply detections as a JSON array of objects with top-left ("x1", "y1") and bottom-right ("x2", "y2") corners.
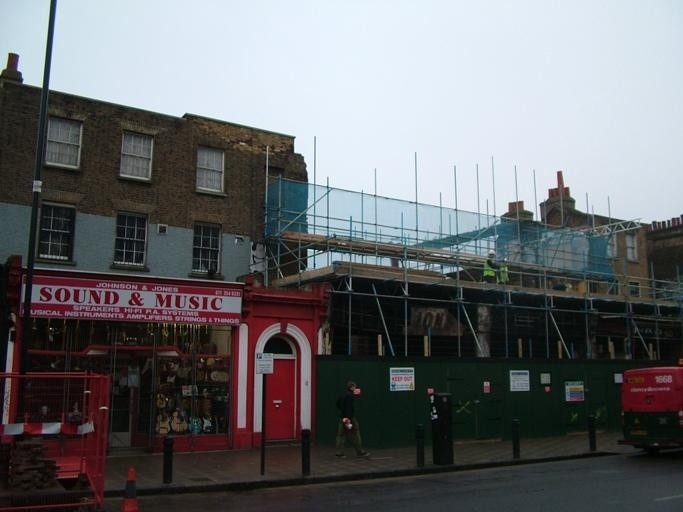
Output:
[
  {"x1": 482, "y1": 250, "x2": 509, "y2": 284},
  {"x1": 334, "y1": 381, "x2": 371, "y2": 459}
]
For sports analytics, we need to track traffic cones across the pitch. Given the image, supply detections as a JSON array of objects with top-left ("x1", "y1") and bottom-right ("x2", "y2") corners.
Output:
[{"x1": 121, "y1": 465, "x2": 142, "y2": 512}]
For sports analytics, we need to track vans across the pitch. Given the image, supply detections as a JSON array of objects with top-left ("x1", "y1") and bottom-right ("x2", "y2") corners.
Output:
[{"x1": 616, "y1": 365, "x2": 683, "y2": 457}]
